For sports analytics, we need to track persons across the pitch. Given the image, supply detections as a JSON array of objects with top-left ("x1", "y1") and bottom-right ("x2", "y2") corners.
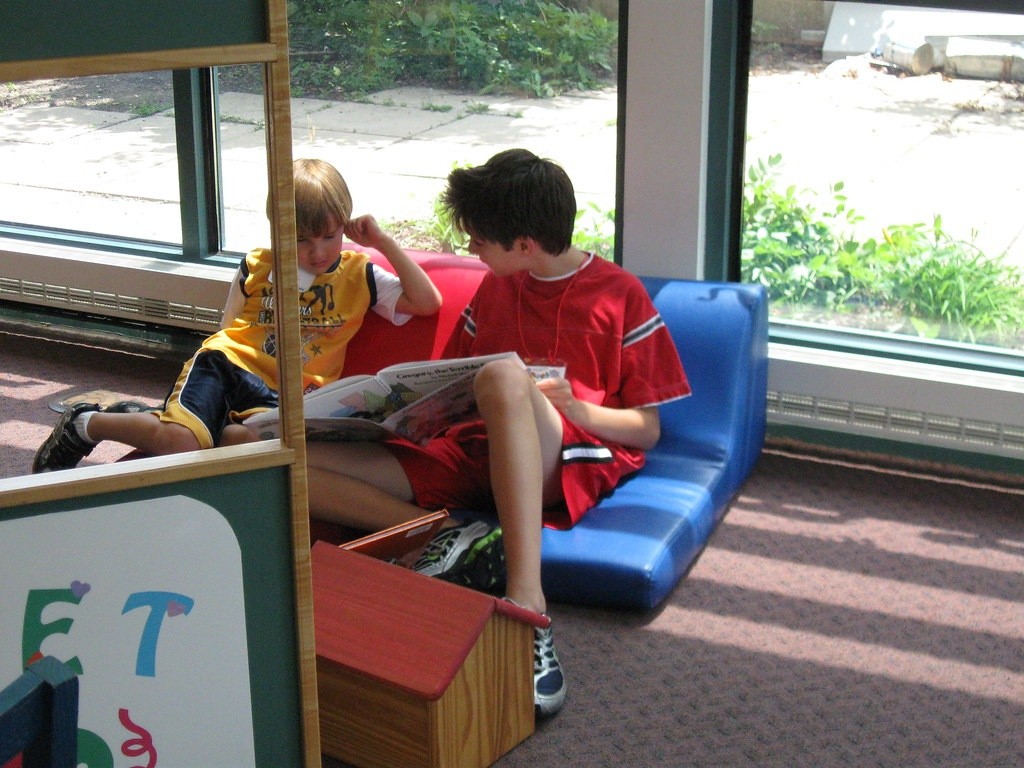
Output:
[
  {"x1": 304, "y1": 148, "x2": 693, "y2": 717},
  {"x1": 31, "y1": 158, "x2": 443, "y2": 484}
]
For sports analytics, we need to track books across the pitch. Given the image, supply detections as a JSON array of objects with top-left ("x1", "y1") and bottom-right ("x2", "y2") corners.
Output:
[{"x1": 240, "y1": 351, "x2": 565, "y2": 446}]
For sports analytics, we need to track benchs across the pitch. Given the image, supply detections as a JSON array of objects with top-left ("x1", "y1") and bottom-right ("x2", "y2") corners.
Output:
[{"x1": 341, "y1": 243, "x2": 767, "y2": 617}]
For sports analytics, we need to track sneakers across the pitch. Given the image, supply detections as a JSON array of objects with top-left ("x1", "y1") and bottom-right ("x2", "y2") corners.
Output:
[
  {"x1": 32, "y1": 400, "x2": 102, "y2": 475},
  {"x1": 104, "y1": 398, "x2": 169, "y2": 413},
  {"x1": 534, "y1": 611, "x2": 568, "y2": 715},
  {"x1": 410, "y1": 518, "x2": 506, "y2": 599}
]
